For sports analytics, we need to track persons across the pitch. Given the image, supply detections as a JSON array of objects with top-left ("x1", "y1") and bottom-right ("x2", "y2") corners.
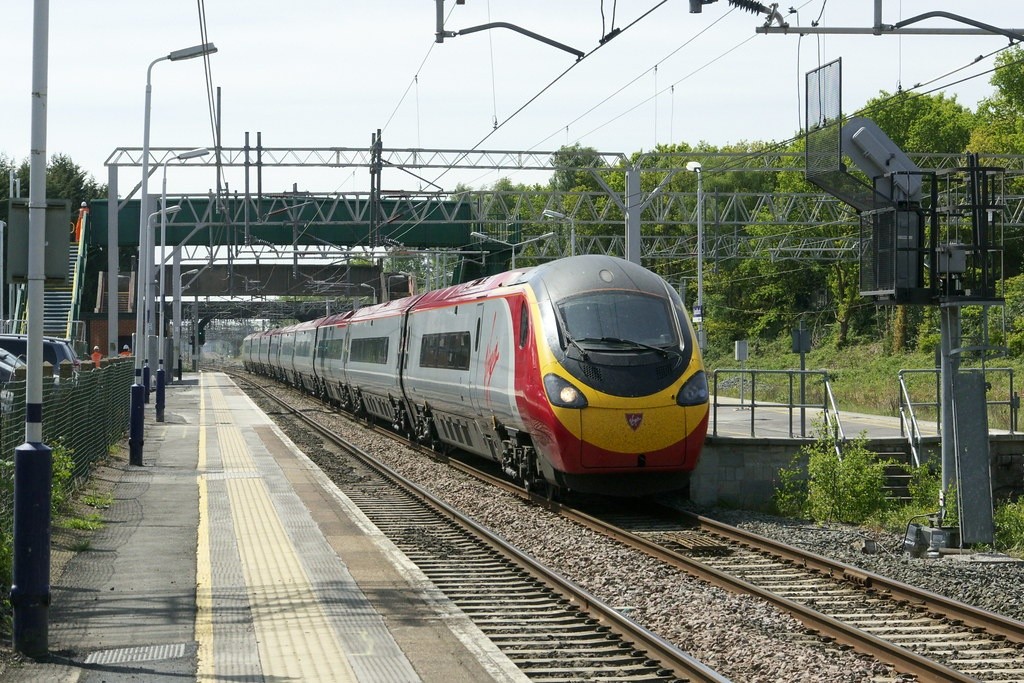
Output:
[
  {"x1": 74, "y1": 202, "x2": 89, "y2": 242},
  {"x1": 121, "y1": 345, "x2": 131, "y2": 356},
  {"x1": 91, "y1": 346, "x2": 103, "y2": 368}
]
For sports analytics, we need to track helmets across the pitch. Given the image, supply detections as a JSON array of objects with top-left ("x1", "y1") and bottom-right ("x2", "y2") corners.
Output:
[
  {"x1": 123, "y1": 345, "x2": 129, "y2": 349},
  {"x1": 94, "y1": 346, "x2": 99, "y2": 350}
]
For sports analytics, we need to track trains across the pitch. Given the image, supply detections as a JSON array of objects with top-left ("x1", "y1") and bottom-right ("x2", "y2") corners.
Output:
[{"x1": 243, "y1": 252, "x2": 710, "y2": 512}]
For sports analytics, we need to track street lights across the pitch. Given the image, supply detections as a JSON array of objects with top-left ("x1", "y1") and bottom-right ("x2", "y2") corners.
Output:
[
  {"x1": 155, "y1": 147, "x2": 209, "y2": 424},
  {"x1": 470, "y1": 230, "x2": 554, "y2": 270},
  {"x1": 684, "y1": 158, "x2": 706, "y2": 362},
  {"x1": 541, "y1": 210, "x2": 576, "y2": 256},
  {"x1": 388, "y1": 274, "x2": 405, "y2": 302},
  {"x1": 130, "y1": 42, "x2": 219, "y2": 466},
  {"x1": 360, "y1": 283, "x2": 376, "y2": 306},
  {"x1": 144, "y1": 203, "x2": 181, "y2": 404}
]
